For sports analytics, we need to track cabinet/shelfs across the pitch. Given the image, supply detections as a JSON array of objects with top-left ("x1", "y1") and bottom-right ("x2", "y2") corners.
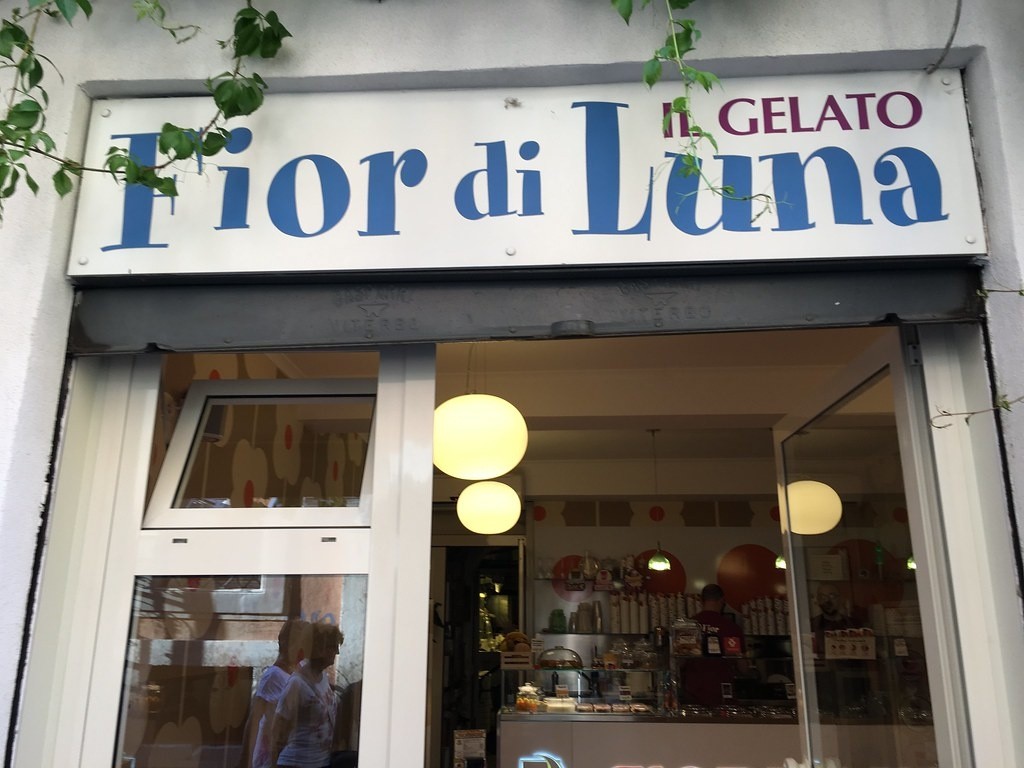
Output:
[{"x1": 530, "y1": 577, "x2": 791, "y2": 694}]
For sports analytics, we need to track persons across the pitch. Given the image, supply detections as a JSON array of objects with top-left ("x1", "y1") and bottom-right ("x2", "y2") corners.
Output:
[
  {"x1": 238, "y1": 619, "x2": 309, "y2": 768},
  {"x1": 273, "y1": 622, "x2": 345, "y2": 768},
  {"x1": 331, "y1": 678, "x2": 362, "y2": 768},
  {"x1": 811, "y1": 582, "x2": 881, "y2": 716},
  {"x1": 678, "y1": 583, "x2": 749, "y2": 706}
]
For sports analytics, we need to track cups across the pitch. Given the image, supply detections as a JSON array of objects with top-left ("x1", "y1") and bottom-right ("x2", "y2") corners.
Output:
[{"x1": 568, "y1": 563, "x2": 584, "y2": 583}]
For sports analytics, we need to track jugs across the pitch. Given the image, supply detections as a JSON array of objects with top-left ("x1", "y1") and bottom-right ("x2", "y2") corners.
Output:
[{"x1": 576, "y1": 602, "x2": 594, "y2": 633}]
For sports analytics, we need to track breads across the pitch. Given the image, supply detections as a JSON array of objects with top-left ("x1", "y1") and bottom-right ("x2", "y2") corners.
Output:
[{"x1": 500, "y1": 632, "x2": 531, "y2": 664}]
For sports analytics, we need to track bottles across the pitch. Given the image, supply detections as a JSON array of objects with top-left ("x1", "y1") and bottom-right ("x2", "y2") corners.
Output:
[
  {"x1": 550, "y1": 609, "x2": 566, "y2": 633},
  {"x1": 569, "y1": 612, "x2": 577, "y2": 633},
  {"x1": 579, "y1": 549, "x2": 601, "y2": 579},
  {"x1": 593, "y1": 600, "x2": 602, "y2": 633}
]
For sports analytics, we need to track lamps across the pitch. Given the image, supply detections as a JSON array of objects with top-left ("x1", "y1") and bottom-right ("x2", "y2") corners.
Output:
[
  {"x1": 457, "y1": 481, "x2": 522, "y2": 536},
  {"x1": 433, "y1": 342, "x2": 529, "y2": 481},
  {"x1": 775, "y1": 551, "x2": 787, "y2": 570},
  {"x1": 907, "y1": 554, "x2": 917, "y2": 569},
  {"x1": 647, "y1": 428, "x2": 671, "y2": 571},
  {"x1": 787, "y1": 480, "x2": 843, "y2": 535}
]
